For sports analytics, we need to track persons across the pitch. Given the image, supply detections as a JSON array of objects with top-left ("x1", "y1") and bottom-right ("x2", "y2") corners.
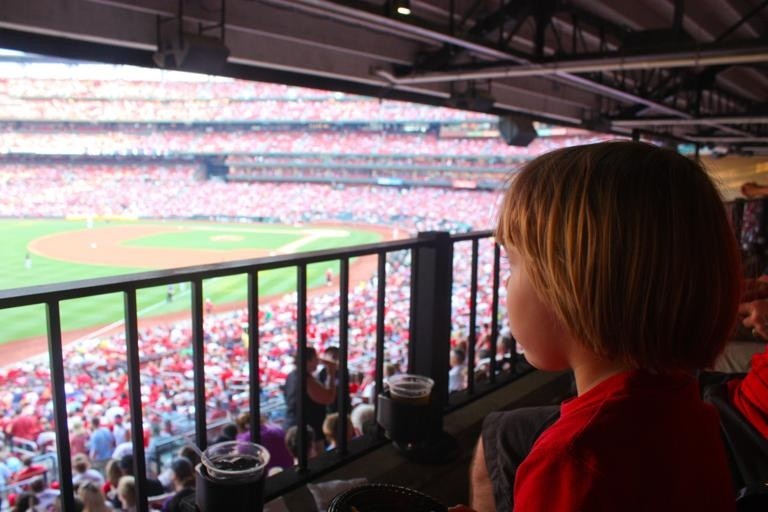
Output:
[
  {"x1": 2, "y1": 46, "x2": 625, "y2": 233},
  {"x1": 496, "y1": 139, "x2": 752, "y2": 512},
  {"x1": 0, "y1": 220, "x2": 624, "y2": 512},
  {"x1": 469, "y1": 274, "x2": 767, "y2": 511},
  {"x1": 731, "y1": 178, "x2": 768, "y2": 282}
]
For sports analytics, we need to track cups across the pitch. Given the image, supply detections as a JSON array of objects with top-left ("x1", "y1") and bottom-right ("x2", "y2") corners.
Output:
[
  {"x1": 202, "y1": 440, "x2": 271, "y2": 480},
  {"x1": 385, "y1": 374, "x2": 433, "y2": 449}
]
